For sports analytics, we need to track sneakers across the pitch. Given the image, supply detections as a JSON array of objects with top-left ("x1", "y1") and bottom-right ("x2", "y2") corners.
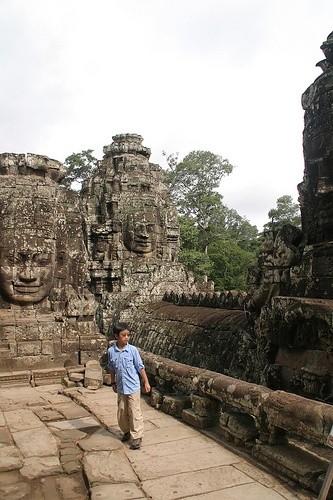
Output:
[
  {"x1": 121, "y1": 433, "x2": 131, "y2": 442},
  {"x1": 130, "y1": 438, "x2": 143, "y2": 449}
]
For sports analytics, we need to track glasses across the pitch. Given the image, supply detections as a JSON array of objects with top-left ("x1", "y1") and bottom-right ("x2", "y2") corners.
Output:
[{"x1": 119, "y1": 333, "x2": 131, "y2": 337}]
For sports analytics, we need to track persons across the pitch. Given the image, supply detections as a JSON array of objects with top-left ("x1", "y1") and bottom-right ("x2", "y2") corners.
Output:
[
  {"x1": 108, "y1": 322, "x2": 151, "y2": 450},
  {"x1": 125, "y1": 212, "x2": 156, "y2": 253},
  {"x1": 0, "y1": 235, "x2": 55, "y2": 304}
]
